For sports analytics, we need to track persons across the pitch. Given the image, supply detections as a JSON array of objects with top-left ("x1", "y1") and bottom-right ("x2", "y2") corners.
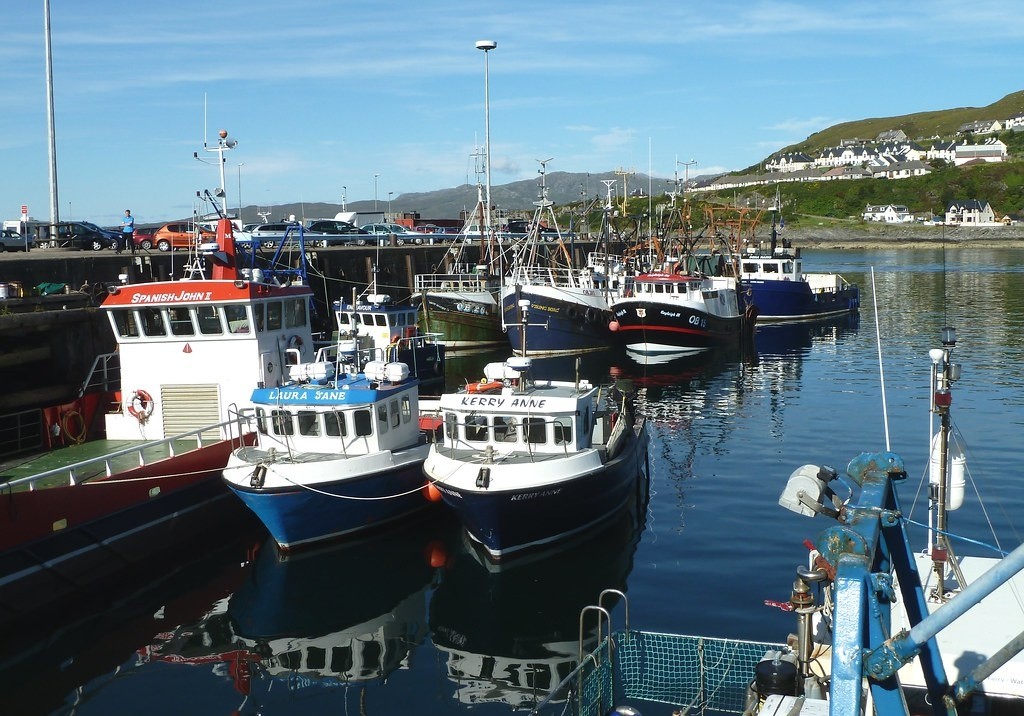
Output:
[{"x1": 116, "y1": 209, "x2": 135, "y2": 255}]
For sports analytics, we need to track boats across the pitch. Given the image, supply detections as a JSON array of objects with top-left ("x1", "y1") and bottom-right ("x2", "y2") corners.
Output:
[
  {"x1": 498, "y1": 210, "x2": 654, "y2": 360},
  {"x1": 610, "y1": 270, "x2": 762, "y2": 366},
  {"x1": 423, "y1": 300, "x2": 651, "y2": 572},
  {"x1": 427, "y1": 474, "x2": 646, "y2": 708},
  {"x1": 229, "y1": 509, "x2": 445, "y2": 716},
  {"x1": 222, "y1": 287, "x2": 445, "y2": 550},
  {"x1": 408, "y1": 146, "x2": 510, "y2": 347},
  {"x1": 738, "y1": 183, "x2": 860, "y2": 325},
  {"x1": 0, "y1": 92, "x2": 445, "y2": 585}
]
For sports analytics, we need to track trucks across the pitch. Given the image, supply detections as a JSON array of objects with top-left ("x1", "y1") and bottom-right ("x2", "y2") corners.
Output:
[{"x1": 334, "y1": 211, "x2": 384, "y2": 230}]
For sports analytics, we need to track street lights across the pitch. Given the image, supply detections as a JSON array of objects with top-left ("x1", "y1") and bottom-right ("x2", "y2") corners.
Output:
[
  {"x1": 343, "y1": 174, "x2": 379, "y2": 211},
  {"x1": 237, "y1": 163, "x2": 244, "y2": 220},
  {"x1": 388, "y1": 191, "x2": 393, "y2": 223}
]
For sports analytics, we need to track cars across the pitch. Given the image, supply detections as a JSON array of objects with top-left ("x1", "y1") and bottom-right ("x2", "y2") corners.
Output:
[
  {"x1": 76, "y1": 221, "x2": 123, "y2": 250},
  {"x1": 503, "y1": 221, "x2": 561, "y2": 242},
  {"x1": 57, "y1": 223, "x2": 113, "y2": 251},
  {"x1": 250, "y1": 222, "x2": 330, "y2": 249},
  {"x1": 360, "y1": 224, "x2": 405, "y2": 246},
  {"x1": 389, "y1": 223, "x2": 424, "y2": 245},
  {"x1": 308, "y1": 221, "x2": 374, "y2": 248},
  {"x1": 428, "y1": 227, "x2": 458, "y2": 244},
  {"x1": 463, "y1": 225, "x2": 505, "y2": 244},
  {"x1": 132, "y1": 227, "x2": 162, "y2": 250},
  {"x1": 0, "y1": 230, "x2": 31, "y2": 253},
  {"x1": 152, "y1": 221, "x2": 217, "y2": 252}
]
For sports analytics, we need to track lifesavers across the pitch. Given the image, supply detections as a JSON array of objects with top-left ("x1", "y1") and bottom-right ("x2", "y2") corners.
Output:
[
  {"x1": 464, "y1": 380, "x2": 504, "y2": 393},
  {"x1": 126, "y1": 389, "x2": 155, "y2": 420}
]
